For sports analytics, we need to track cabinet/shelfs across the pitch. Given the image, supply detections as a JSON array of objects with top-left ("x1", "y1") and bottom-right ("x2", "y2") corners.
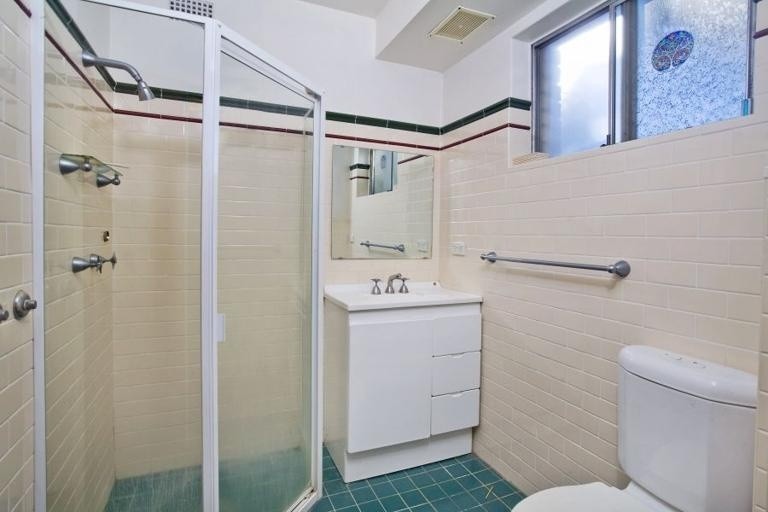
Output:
[{"x1": 321, "y1": 313, "x2": 484, "y2": 453}]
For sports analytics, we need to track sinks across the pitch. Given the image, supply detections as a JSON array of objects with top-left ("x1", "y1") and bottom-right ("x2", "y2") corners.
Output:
[{"x1": 366, "y1": 292, "x2": 430, "y2": 302}]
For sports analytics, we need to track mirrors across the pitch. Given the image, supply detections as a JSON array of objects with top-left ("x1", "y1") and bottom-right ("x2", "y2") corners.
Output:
[{"x1": 329, "y1": 142, "x2": 436, "y2": 261}]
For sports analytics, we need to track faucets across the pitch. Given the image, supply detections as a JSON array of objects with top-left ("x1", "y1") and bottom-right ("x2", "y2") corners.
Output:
[{"x1": 384, "y1": 273, "x2": 402, "y2": 293}]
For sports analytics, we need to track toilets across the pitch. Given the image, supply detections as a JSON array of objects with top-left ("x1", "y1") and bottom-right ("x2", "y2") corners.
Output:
[{"x1": 511, "y1": 346, "x2": 761, "y2": 512}]
